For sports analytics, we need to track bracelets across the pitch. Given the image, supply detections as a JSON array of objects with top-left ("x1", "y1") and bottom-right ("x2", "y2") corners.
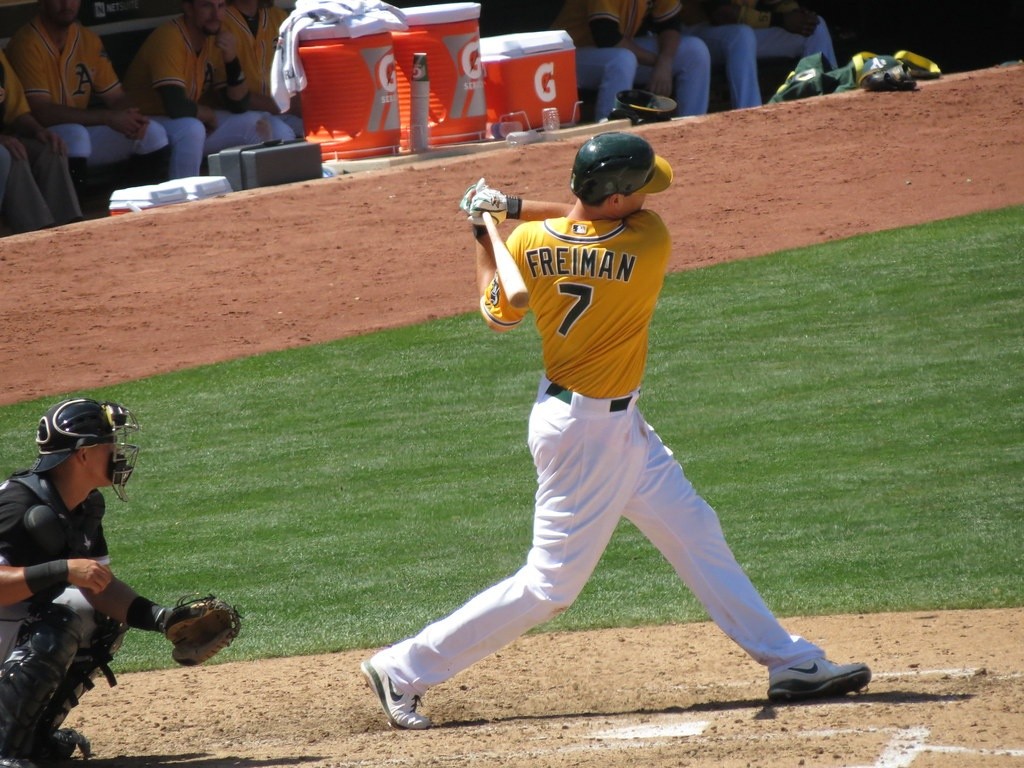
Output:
[{"x1": 153, "y1": 608, "x2": 166, "y2": 631}]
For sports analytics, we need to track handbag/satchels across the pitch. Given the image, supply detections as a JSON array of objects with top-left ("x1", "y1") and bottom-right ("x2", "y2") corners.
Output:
[{"x1": 768, "y1": 50, "x2": 942, "y2": 102}]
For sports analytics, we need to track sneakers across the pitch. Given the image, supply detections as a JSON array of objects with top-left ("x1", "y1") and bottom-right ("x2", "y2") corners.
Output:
[
  {"x1": 359, "y1": 658, "x2": 429, "y2": 730},
  {"x1": 767, "y1": 656, "x2": 871, "y2": 702}
]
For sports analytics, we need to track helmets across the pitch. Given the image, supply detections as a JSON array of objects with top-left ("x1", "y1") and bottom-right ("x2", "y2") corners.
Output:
[
  {"x1": 570, "y1": 132, "x2": 673, "y2": 204},
  {"x1": 858, "y1": 55, "x2": 897, "y2": 89},
  {"x1": 615, "y1": 89, "x2": 678, "y2": 117}
]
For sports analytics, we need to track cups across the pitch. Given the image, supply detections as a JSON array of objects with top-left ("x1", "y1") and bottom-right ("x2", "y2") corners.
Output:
[
  {"x1": 324, "y1": 160, "x2": 343, "y2": 177},
  {"x1": 410, "y1": 53, "x2": 430, "y2": 154}
]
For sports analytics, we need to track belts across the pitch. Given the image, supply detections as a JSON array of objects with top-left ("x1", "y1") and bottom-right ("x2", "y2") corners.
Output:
[{"x1": 546, "y1": 384, "x2": 633, "y2": 411}]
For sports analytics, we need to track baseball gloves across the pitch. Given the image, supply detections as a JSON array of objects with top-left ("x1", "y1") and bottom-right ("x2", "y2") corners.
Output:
[{"x1": 164, "y1": 598, "x2": 242, "y2": 666}]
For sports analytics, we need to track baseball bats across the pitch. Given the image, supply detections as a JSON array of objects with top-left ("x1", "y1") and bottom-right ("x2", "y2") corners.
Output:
[{"x1": 481, "y1": 210, "x2": 531, "y2": 309}]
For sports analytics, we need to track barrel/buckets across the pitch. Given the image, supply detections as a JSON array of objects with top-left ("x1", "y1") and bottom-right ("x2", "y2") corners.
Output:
[
  {"x1": 298, "y1": 20, "x2": 400, "y2": 163},
  {"x1": 389, "y1": 1, "x2": 487, "y2": 149}
]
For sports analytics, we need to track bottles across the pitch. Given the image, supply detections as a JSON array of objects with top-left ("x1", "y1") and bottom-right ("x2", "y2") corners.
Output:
[
  {"x1": 322, "y1": 167, "x2": 336, "y2": 178},
  {"x1": 506, "y1": 130, "x2": 542, "y2": 147},
  {"x1": 542, "y1": 107, "x2": 561, "y2": 133},
  {"x1": 486, "y1": 121, "x2": 524, "y2": 140}
]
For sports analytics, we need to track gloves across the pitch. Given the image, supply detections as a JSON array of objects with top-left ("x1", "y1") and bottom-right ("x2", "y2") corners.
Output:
[{"x1": 459, "y1": 178, "x2": 522, "y2": 238}]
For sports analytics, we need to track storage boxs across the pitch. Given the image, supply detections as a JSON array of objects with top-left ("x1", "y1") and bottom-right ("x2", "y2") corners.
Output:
[
  {"x1": 107, "y1": 184, "x2": 195, "y2": 217},
  {"x1": 206, "y1": 139, "x2": 324, "y2": 191},
  {"x1": 160, "y1": 175, "x2": 233, "y2": 204},
  {"x1": 482, "y1": 29, "x2": 584, "y2": 130}
]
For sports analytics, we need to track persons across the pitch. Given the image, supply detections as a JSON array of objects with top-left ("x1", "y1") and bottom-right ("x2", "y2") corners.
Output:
[
  {"x1": 361, "y1": 126, "x2": 871, "y2": 729},
  {"x1": 0, "y1": 0, "x2": 290, "y2": 236},
  {"x1": 547, "y1": 0, "x2": 838, "y2": 124},
  {"x1": 0, "y1": 396, "x2": 244, "y2": 768}
]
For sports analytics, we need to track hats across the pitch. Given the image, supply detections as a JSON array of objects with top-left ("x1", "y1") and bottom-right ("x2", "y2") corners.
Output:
[{"x1": 32, "y1": 399, "x2": 115, "y2": 472}]
